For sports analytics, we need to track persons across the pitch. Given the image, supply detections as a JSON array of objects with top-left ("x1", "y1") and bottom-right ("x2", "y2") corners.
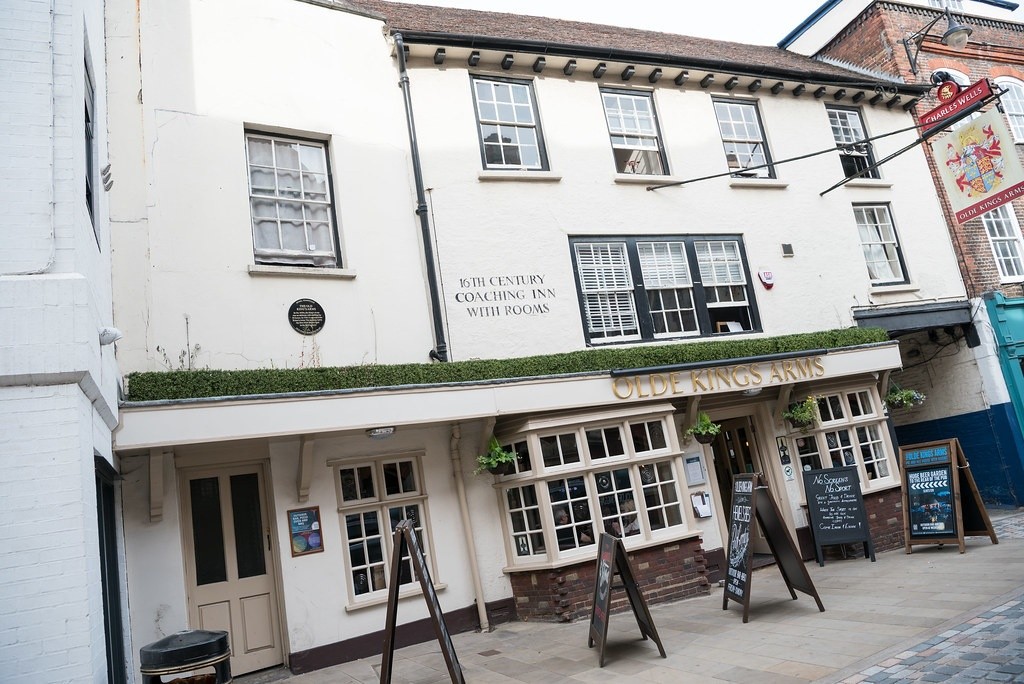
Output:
[
  {"x1": 613, "y1": 500, "x2": 640, "y2": 536},
  {"x1": 553, "y1": 507, "x2": 592, "y2": 545}
]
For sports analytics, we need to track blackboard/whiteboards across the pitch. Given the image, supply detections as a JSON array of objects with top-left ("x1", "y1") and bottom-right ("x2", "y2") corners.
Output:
[
  {"x1": 403, "y1": 519, "x2": 466, "y2": 684},
  {"x1": 589, "y1": 533, "x2": 618, "y2": 646},
  {"x1": 725, "y1": 473, "x2": 759, "y2": 604},
  {"x1": 802, "y1": 465, "x2": 869, "y2": 546}
]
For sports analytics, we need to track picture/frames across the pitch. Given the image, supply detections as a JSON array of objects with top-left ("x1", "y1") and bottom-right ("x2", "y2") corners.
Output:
[{"x1": 286, "y1": 505, "x2": 325, "y2": 557}]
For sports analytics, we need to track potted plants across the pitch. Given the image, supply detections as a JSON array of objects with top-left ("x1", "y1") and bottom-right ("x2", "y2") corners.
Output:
[
  {"x1": 778, "y1": 388, "x2": 826, "y2": 436},
  {"x1": 683, "y1": 409, "x2": 721, "y2": 444},
  {"x1": 880, "y1": 382, "x2": 927, "y2": 414},
  {"x1": 466, "y1": 434, "x2": 523, "y2": 475}
]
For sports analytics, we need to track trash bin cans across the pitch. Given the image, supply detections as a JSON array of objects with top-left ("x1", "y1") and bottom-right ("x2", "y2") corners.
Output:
[{"x1": 140, "y1": 628, "x2": 232, "y2": 684}]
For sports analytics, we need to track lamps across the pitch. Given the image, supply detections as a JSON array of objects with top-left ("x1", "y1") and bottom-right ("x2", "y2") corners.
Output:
[
  {"x1": 100, "y1": 161, "x2": 111, "y2": 176},
  {"x1": 103, "y1": 179, "x2": 114, "y2": 191},
  {"x1": 365, "y1": 425, "x2": 396, "y2": 441},
  {"x1": 902, "y1": 6, "x2": 973, "y2": 76},
  {"x1": 102, "y1": 172, "x2": 112, "y2": 184}
]
